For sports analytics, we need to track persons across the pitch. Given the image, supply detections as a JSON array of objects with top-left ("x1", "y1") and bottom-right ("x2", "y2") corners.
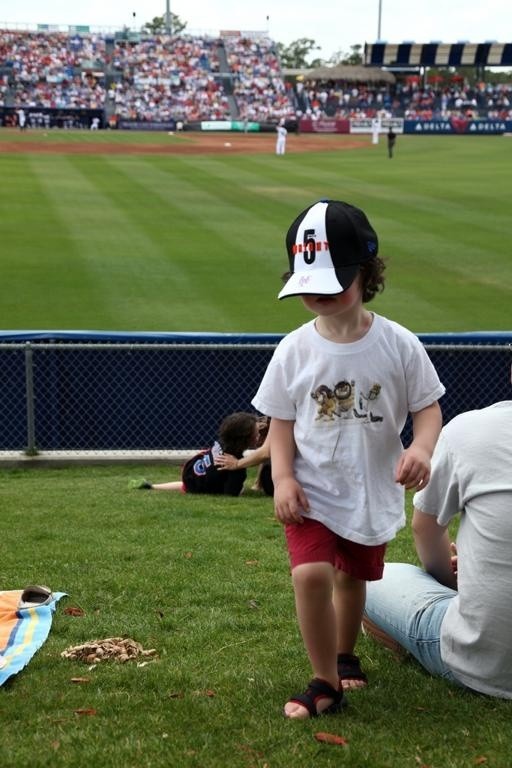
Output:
[
  {"x1": 372, "y1": 120, "x2": 380, "y2": 145},
  {"x1": 1, "y1": 34, "x2": 511, "y2": 132},
  {"x1": 360, "y1": 400, "x2": 512, "y2": 702},
  {"x1": 275, "y1": 124, "x2": 288, "y2": 156},
  {"x1": 387, "y1": 126, "x2": 397, "y2": 159},
  {"x1": 127, "y1": 411, "x2": 261, "y2": 497},
  {"x1": 253, "y1": 199, "x2": 446, "y2": 719},
  {"x1": 213, "y1": 415, "x2": 274, "y2": 497}
]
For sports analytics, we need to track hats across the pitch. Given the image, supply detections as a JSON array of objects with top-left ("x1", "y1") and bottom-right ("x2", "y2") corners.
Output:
[{"x1": 277, "y1": 200, "x2": 380, "y2": 301}]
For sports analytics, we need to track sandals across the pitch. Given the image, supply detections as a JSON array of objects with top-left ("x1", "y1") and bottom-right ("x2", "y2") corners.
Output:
[
  {"x1": 338, "y1": 652, "x2": 368, "y2": 691},
  {"x1": 283, "y1": 676, "x2": 348, "y2": 722}
]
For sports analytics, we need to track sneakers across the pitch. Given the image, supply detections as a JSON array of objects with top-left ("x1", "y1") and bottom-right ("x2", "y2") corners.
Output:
[{"x1": 129, "y1": 479, "x2": 152, "y2": 491}]
